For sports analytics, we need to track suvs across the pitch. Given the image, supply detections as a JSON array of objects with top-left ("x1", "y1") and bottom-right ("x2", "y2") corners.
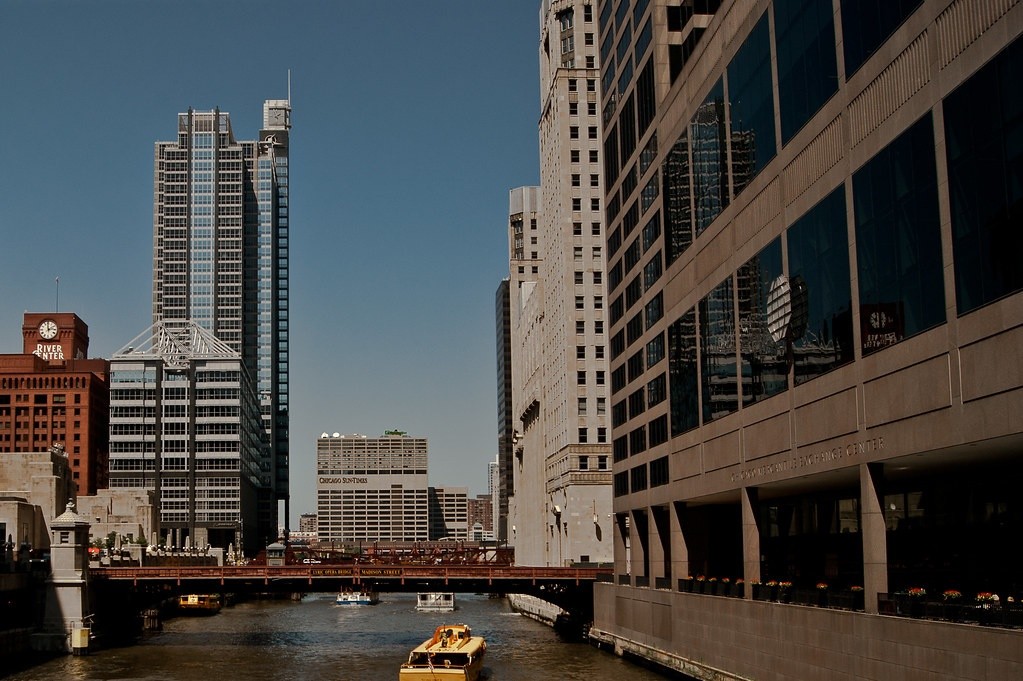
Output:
[{"x1": 302, "y1": 558, "x2": 322, "y2": 564}]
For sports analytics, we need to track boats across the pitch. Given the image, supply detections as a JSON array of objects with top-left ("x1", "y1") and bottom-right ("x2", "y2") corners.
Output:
[
  {"x1": 414, "y1": 591, "x2": 456, "y2": 613},
  {"x1": 177, "y1": 593, "x2": 222, "y2": 616},
  {"x1": 398, "y1": 622, "x2": 487, "y2": 681},
  {"x1": 335, "y1": 585, "x2": 379, "y2": 605}
]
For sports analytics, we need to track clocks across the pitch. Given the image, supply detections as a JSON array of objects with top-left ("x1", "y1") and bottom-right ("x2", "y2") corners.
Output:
[{"x1": 38, "y1": 318, "x2": 58, "y2": 339}]
